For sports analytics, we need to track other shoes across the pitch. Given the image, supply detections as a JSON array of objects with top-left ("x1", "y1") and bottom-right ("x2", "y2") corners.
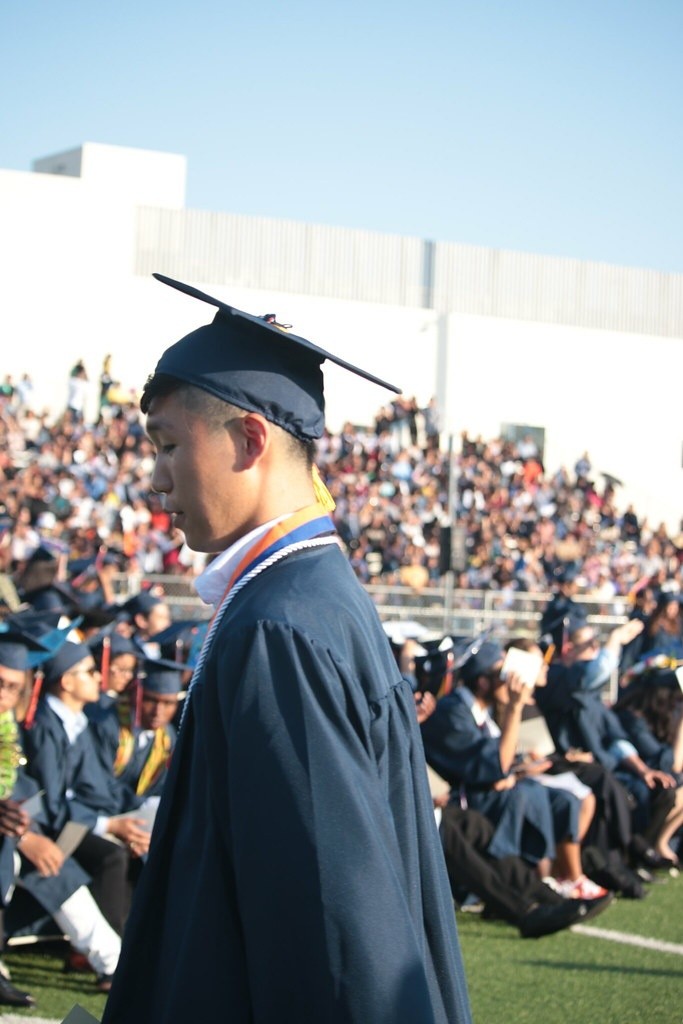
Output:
[
  {"x1": 581, "y1": 834, "x2": 683, "y2": 898},
  {"x1": 97, "y1": 973, "x2": 113, "y2": 990},
  {"x1": 521, "y1": 896, "x2": 610, "y2": 939}
]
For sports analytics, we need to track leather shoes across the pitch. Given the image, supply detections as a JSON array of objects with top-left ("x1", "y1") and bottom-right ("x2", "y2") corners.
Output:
[{"x1": 0, "y1": 974, "x2": 34, "y2": 1007}]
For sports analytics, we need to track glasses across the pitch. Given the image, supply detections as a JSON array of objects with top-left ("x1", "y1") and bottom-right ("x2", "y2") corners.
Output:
[{"x1": 72, "y1": 666, "x2": 98, "y2": 678}]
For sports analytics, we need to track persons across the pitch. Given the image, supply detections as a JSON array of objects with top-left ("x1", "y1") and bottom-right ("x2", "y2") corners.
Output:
[{"x1": 0, "y1": 273, "x2": 683, "y2": 1024}]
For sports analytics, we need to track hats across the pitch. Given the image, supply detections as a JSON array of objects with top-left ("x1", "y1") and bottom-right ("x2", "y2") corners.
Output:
[
  {"x1": 387, "y1": 590, "x2": 683, "y2": 700},
  {"x1": 153, "y1": 273, "x2": 402, "y2": 511},
  {"x1": 0, "y1": 581, "x2": 198, "y2": 731}
]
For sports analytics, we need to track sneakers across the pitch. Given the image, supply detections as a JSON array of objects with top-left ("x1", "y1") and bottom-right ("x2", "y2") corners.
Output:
[{"x1": 543, "y1": 875, "x2": 608, "y2": 900}]
